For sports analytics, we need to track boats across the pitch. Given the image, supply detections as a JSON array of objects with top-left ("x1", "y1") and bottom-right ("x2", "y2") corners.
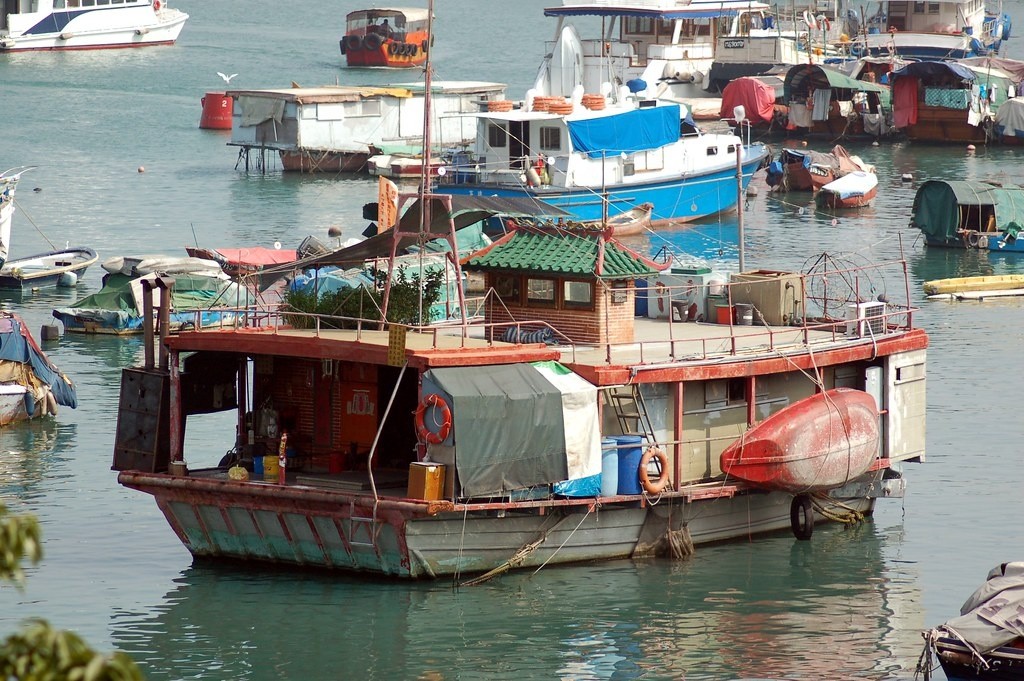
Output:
[
  {"x1": 921, "y1": 560, "x2": 1024, "y2": 681},
  {"x1": 585, "y1": 202, "x2": 654, "y2": 237},
  {"x1": 223, "y1": 81, "x2": 508, "y2": 172},
  {"x1": 923, "y1": 274, "x2": 1024, "y2": 300},
  {"x1": 720, "y1": 386, "x2": 880, "y2": 492},
  {"x1": 110, "y1": 1, "x2": 930, "y2": 575},
  {"x1": 765, "y1": 144, "x2": 879, "y2": 208},
  {"x1": 0, "y1": 309, "x2": 78, "y2": 429},
  {"x1": 0, "y1": 0, "x2": 190, "y2": 53},
  {"x1": 340, "y1": 7, "x2": 435, "y2": 69},
  {"x1": 0, "y1": 165, "x2": 99, "y2": 288},
  {"x1": 52, "y1": 181, "x2": 579, "y2": 336},
  {"x1": 910, "y1": 178, "x2": 1024, "y2": 251},
  {"x1": 534, "y1": 0, "x2": 1024, "y2": 150},
  {"x1": 367, "y1": 153, "x2": 441, "y2": 179}
]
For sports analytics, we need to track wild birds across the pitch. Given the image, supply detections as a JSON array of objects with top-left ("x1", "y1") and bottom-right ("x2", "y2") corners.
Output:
[{"x1": 217, "y1": 71, "x2": 239, "y2": 84}]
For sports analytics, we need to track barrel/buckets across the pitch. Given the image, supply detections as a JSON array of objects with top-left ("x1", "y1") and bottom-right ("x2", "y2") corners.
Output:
[
  {"x1": 715, "y1": 305, "x2": 735, "y2": 325},
  {"x1": 264, "y1": 457, "x2": 278, "y2": 482},
  {"x1": 254, "y1": 458, "x2": 264, "y2": 474},
  {"x1": 672, "y1": 300, "x2": 688, "y2": 322},
  {"x1": 736, "y1": 303, "x2": 753, "y2": 325},
  {"x1": 707, "y1": 295, "x2": 728, "y2": 323},
  {"x1": 607, "y1": 436, "x2": 642, "y2": 495},
  {"x1": 601, "y1": 441, "x2": 618, "y2": 496}
]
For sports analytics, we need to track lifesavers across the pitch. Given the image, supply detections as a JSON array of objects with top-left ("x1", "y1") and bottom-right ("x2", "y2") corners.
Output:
[
  {"x1": 398, "y1": 44, "x2": 406, "y2": 55},
  {"x1": 154, "y1": 0, "x2": 160, "y2": 10},
  {"x1": 406, "y1": 44, "x2": 412, "y2": 56},
  {"x1": 548, "y1": 103, "x2": 574, "y2": 115},
  {"x1": 638, "y1": 447, "x2": 670, "y2": 495},
  {"x1": 412, "y1": 45, "x2": 417, "y2": 56},
  {"x1": 390, "y1": 42, "x2": 398, "y2": 55},
  {"x1": 364, "y1": 32, "x2": 382, "y2": 51},
  {"x1": 431, "y1": 34, "x2": 434, "y2": 48},
  {"x1": 850, "y1": 41, "x2": 865, "y2": 57},
  {"x1": 968, "y1": 229, "x2": 980, "y2": 247},
  {"x1": 347, "y1": 35, "x2": 361, "y2": 51},
  {"x1": 342, "y1": 36, "x2": 348, "y2": 48},
  {"x1": 790, "y1": 495, "x2": 814, "y2": 541},
  {"x1": 803, "y1": 10, "x2": 816, "y2": 29},
  {"x1": 816, "y1": 15, "x2": 830, "y2": 32},
  {"x1": 415, "y1": 395, "x2": 452, "y2": 444},
  {"x1": 532, "y1": 97, "x2": 565, "y2": 112},
  {"x1": 340, "y1": 40, "x2": 345, "y2": 54},
  {"x1": 486, "y1": 101, "x2": 513, "y2": 112},
  {"x1": 582, "y1": 93, "x2": 605, "y2": 110},
  {"x1": 422, "y1": 40, "x2": 427, "y2": 52}
]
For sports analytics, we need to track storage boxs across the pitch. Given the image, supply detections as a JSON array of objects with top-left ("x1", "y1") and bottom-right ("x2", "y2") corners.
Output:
[
  {"x1": 706, "y1": 295, "x2": 735, "y2": 325},
  {"x1": 407, "y1": 461, "x2": 446, "y2": 501}
]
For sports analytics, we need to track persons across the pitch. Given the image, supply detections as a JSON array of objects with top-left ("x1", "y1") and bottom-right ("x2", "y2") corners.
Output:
[{"x1": 379, "y1": 19, "x2": 392, "y2": 32}]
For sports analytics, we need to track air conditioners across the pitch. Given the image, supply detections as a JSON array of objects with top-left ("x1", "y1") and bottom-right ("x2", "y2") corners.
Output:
[{"x1": 845, "y1": 301, "x2": 886, "y2": 337}]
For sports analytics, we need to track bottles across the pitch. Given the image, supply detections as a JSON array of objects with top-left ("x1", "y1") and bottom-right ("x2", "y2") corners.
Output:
[{"x1": 247, "y1": 412, "x2": 255, "y2": 444}]
